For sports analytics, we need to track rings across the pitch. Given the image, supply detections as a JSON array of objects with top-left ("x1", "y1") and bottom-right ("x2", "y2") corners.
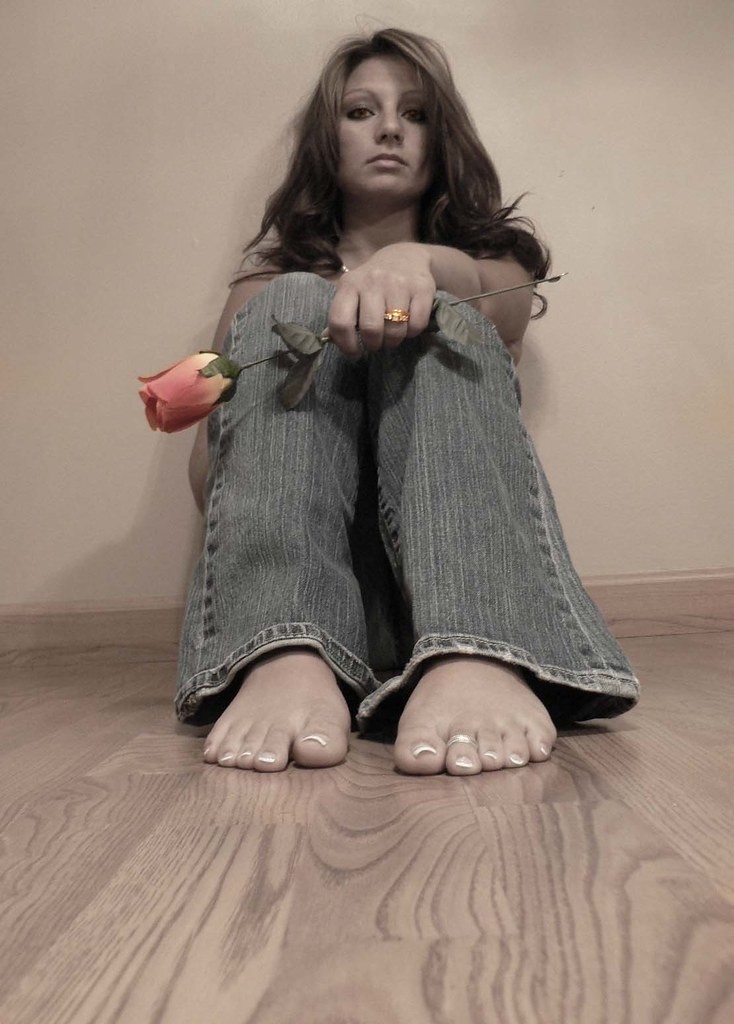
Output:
[{"x1": 385, "y1": 310, "x2": 412, "y2": 322}]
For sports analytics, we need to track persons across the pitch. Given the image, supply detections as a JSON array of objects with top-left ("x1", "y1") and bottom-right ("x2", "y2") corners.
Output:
[{"x1": 172, "y1": 26, "x2": 639, "y2": 778}]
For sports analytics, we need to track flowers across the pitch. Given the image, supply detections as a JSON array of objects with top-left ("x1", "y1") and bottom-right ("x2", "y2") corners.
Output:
[{"x1": 137, "y1": 272, "x2": 570, "y2": 434}]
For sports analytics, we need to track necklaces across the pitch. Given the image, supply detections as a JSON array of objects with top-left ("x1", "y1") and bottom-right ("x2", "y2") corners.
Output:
[{"x1": 341, "y1": 264, "x2": 350, "y2": 272}]
